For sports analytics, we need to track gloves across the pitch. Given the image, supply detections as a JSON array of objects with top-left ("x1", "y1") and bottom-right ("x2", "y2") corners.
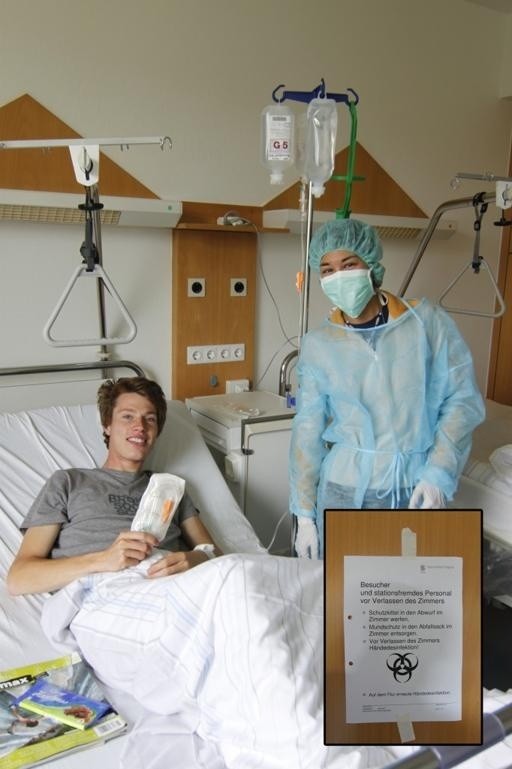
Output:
[
  {"x1": 296, "y1": 515, "x2": 320, "y2": 557},
  {"x1": 409, "y1": 482, "x2": 444, "y2": 510}
]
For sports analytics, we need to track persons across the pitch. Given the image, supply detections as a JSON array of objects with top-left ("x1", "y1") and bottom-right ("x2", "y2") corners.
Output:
[
  {"x1": 289, "y1": 218, "x2": 488, "y2": 559},
  {"x1": 6, "y1": 375, "x2": 223, "y2": 603}
]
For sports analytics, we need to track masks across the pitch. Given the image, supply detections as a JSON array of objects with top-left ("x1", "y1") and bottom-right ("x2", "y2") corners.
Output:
[{"x1": 321, "y1": 267, "x2": 376, "y2": 319}]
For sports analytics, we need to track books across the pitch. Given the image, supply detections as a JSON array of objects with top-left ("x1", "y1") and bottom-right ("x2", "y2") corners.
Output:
[{"x1": 0, "y1": 652, "x2": 128, "y2": 769}]
